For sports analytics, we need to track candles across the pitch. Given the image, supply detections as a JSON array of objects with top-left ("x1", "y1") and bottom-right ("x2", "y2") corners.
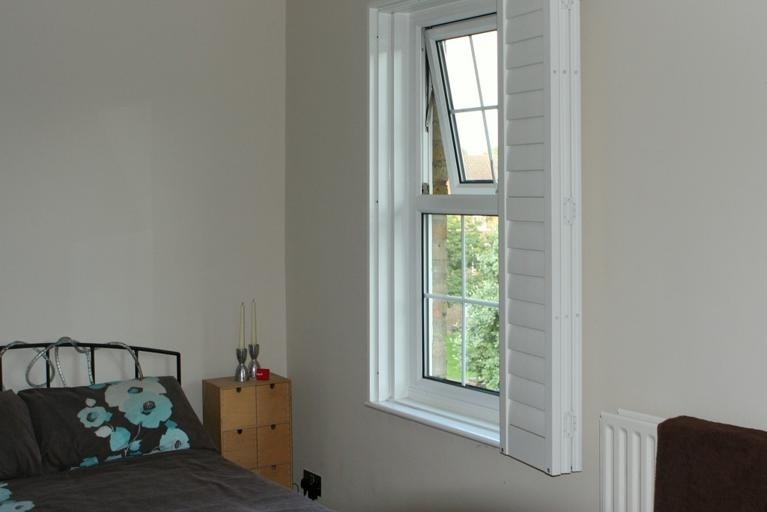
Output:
[{"x1": 238, "y1": 298, "x2": 256, "y2": 348}]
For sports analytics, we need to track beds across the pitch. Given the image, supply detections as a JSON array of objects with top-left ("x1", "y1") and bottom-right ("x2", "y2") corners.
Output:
[{"x1": 1, "y1": 339, "x2": 340, "y2": 512}]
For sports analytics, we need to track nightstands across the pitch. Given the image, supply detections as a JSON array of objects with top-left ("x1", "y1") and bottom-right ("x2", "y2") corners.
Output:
[{"x1": 200, "y1": 374, "x2": 295, "y2": 490}]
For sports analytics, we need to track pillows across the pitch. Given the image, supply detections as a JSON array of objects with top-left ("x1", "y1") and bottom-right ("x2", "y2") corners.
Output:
[{"x1": 0, "y1": 374, "x2": 216, "y2": 482}]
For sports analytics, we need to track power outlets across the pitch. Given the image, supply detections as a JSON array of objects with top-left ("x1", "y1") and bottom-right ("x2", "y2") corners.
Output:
[{"x1": 300, "y1": 468, "x2": 323, "y2": 500}]
